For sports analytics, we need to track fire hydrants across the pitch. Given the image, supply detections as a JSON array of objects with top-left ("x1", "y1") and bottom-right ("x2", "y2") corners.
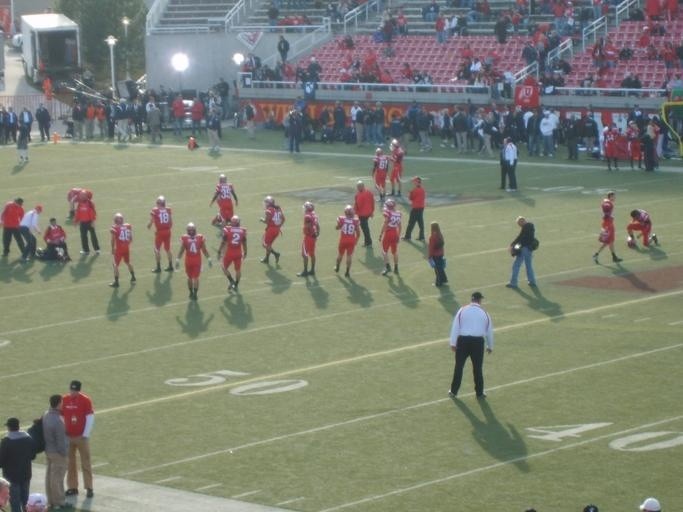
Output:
[{"x1": 49, "y1": 131, "x2": 60, "y2": 144}]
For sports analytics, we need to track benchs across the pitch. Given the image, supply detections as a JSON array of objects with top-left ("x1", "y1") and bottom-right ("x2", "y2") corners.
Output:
[{"x1": 155, "y1": 1, "x2": 623, "y2": 35}]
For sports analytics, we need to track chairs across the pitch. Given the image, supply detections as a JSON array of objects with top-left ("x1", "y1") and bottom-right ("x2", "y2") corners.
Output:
[{"x1": 282, "y1": 0, "x2": 682, "y2": 97}]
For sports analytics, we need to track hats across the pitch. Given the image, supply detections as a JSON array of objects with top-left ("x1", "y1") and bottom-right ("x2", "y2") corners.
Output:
[
  {"x1": 471, "y1": 292, "x2": 483, "y2": 300},
  {"x1": 27, "y1": 493, "x2": 47, "y2": 506},
  {"x1": 71, "y1": 380, "x2": 81, "y2": 391}
]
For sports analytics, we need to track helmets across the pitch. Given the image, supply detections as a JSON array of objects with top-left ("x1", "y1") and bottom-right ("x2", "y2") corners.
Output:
[
  {"x1": 220, "y1": 174, "x2": 226, "y2": 183},
  {"x1": 156, "y1": 196, "x2": 166, "y2": 209},
  {"x1": 231, "y1": 216, "x2": 240, "y2": 227},
  {"x1": 187, "y1": 223, "x2": 196, "y2": 235},
  {"x1": 303, "y1": 201, "x2": 314, "y2": 213},
  {"x1": 264, "y1": 196, "x2": 274, "y2": 207},
  {"x1": 114, "y1": 213, "x2": 124, "y2": 224},
  {"x1": 344, "y1": 205, "x2": 354, "y2": 218},
  {"x1": 78, "y1": 190, "x2": 88, "y2": 200},
  {"x1": 384, "y1": 199, "x2": 396, "y2": 211}
]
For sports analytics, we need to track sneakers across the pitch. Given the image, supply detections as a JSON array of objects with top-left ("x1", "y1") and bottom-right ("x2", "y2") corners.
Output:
[
  {"x1": 506, "y1": 283, "x2": 517, "y2": 289},
  {"x1": 613, "y1": 256, "x2": 622, "y2": 262},
  {"x1": 109, "y1": 283, "x2": 120, "y2": 287},
  {"x1": 653, "y1": 234, "x2": 657, "y2": 244},
  {"x1": 308, "y1": 270, "x2": 315, "y2": 275},
  {"x1": 152, "y1": 268, "x2": 162, "y2": 272},
  {"x1": 96, "y1": 250, "x2": 101, "y2": 255},
  {"x1": 164, "y1": 267, "x2": 174, "y2": 271},
  {"x1": 228, "y1": 280, "x2": 235, "y2": 290},
  {"x1": 65, "y1": 488, "x2": 79, "y2": 496},
  {"x1": 193, "y1": 296, "x2": 197, "y2": 300},
  {"x1": 275, "y1": 253, "x2": 280, "y2": 264},
  {"x1": 334, "y1": 266, "x2": 339, "y2": 272},
  {"x1": 131, "y1": 277, "x2": 136, "y2": 281},
  {"x1": 297, "y1": 271, "x2": 308, "y2": 276},
  {"x1": 345, "y1": 273, "x2": 349, "y2": 278},
  {"x1": 189, "y1": 292, "x2": 194, "y2": 297},
  {"x1": 87, "y1": 489, "x2": 94, "y2": 498},
  {"x1": 382, "y1": 266, "x2": 391, "y2": 275},
  {"x1": 80, "y1": 251, "x2": 90, "y2": 255},
  {"x1": 261, "y1": 259, "x2": 269, "y2": 264},
  {"x1": 233, "y1": 285, "x2": 238, "y2": 292},
  {"x1": 394, "y1": 269, "x2": 398, "y2": 274},
  {"x1": 591, "y1": 254, "x2": 599, "y2": 263}
]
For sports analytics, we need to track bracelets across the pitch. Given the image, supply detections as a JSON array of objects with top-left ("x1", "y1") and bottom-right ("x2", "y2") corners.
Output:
[
  {"x1": 207, "y1": 257, "x2": 211, "y2": 262},
  {"x1": 243, "y1": 251, "x2": 247, "y2": 256},
  {"x1": 217, "y1": 250, "x2": 220, "y2": 255},
  {"x1": 176, "y1": 258, "x2": 179, "y2": 263}
]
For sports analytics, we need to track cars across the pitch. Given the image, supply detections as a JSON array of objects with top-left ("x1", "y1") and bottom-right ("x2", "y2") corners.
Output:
[{"x1": 12, "y1": 33, "x2": 23, "y2": 51}]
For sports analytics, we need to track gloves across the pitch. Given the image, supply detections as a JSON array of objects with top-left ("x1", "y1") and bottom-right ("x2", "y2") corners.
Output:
[
  {"x1": 175, "y1": 259, "x2": 181, "y2": 270},
  {"x1": 208, "y1": 257, "x2": 213, "y2": 268}
]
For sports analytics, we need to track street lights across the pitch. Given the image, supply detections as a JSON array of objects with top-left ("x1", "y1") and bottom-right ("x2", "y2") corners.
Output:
[
  {"x1": 104, "y1": 35, "x2": 117, "y2": 99},
  {"x1": 172, "y1": 52, "x2": 189, "y2": 96},
  {"x1": 233, "y1": 53, "x2": 243, "y2": 72},
  {"x1": 120, "y1": 16, "x2": 134, "y2": 80}
]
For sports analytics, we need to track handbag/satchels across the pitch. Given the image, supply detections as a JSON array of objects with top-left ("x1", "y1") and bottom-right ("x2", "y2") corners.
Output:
[
  {"x1": 28, "y1": 417, "x2": 45, "y2": 452},
  {"x1": 528, "y1": 240, "x2": 538, "y2": 251}
]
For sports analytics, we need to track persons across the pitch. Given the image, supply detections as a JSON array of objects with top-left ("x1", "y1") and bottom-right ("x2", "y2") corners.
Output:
[
  {"x1": 525, "y1": 507, "x2": 537, "y2": 512},
  {"x1": 175, "y1": 222, "x2": 211, "y2": 297},
  {"x1": 504, "y1": 133, "x2": 517, "y2": 192},
  {"x1": 0, "y1": 472, "x2": 11, "y2": 512},
  {"x1": 426, "y1": 221, "x2": 448, "y2": 286},
  {"x1": 333, "y1": 206, "x2": 361, "y2": 279},
  {"x1": 41, "y1": 394, "x2": 75, "y2": 512},
  {"x1": 16, "y1": 204, "x2": 42, "y2": 264},
  {"x1": 146, "y1": 195, "x2": 175, "y2": 274},
  {"x1": 296, "y1": 201, "x2": 320, "y2": 278},
  {"x1": 0, "y1": 76, "x2": 230, "y2": 164},
  {"x1": 0, "y1": 197, "x2": 25, "y2": 258},
  {"x1": 590, "y1": 192, "x2": 622, "y2": 263},
  {"x1": 387, "y1": 138, "x2": 406, "y2": 197},
  {"x1": 448, "y1": 290, "x2": 493, "y2": 399},
  {"x1": 232, "y1": 0, "x2": 681, "y2": 170},
  {"x1": 42, "y1": 217, "x2": 71, "y2": 261},
  {"x1": 72, "y1": 190, "x2": 99, "y2": 255},
  {"x1": 258, "y1": 194, "x2": 285, "y2": 266},
  {"x1": 497, "y1": 138, "x2": 508, "y2": 189},
  {"x1": 378, "y1": 199, "x2": 403, "y2": 276},
  {"x1": 371, "y1": 146, "x2": 389, "y2": 202},
  {"x1": 352, "y1": 181, "x2": 376, "y2": 247},
  {"x1": 60, "y1": 378, "x2": 95, "y2": 498},
  {"x1": 402, "y1": 173, "x2": 426, "y2": 241},
  {"x1": 66, "y1": 188, "x2": 93, "y2": 226},
  {"x1": 215, "y1": 215, "x2": 249, "y2": 292},
  {"x1": 507, "y1": 216, "x2": 540, "y2": 287},
  {"x1": 109, "y1": 214, "x2": 137, "y2": 288},
  {"x1": 626, "y1": 209, "x2": 659, "y2": 247},
  {"x1": 581, "y1": 504, "x2": 600, "y2": 512},
  {"x1": 0, "y1": 418, "x2": 35, "y2": 511},
  {"x1": 210, "y1": 174, "x2": 238, "y2": 219}
]
